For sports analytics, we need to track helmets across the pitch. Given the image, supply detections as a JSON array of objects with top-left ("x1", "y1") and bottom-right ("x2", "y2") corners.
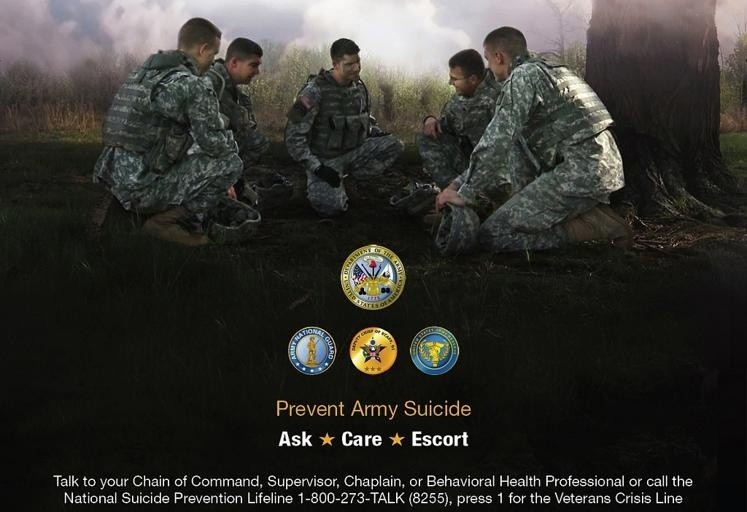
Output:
[
  {"x1": 244, "y1": 166, "x2": 294, "y2": 208},
  {"x1": 435, "y1": 202, "x2": 480, "y2": 263},
  {"x1": 390, "y1": 180, "x2": 442, "y2": 218},
  {"x1": 208, "y1": 195, "x2": 262, "y2": 246}
]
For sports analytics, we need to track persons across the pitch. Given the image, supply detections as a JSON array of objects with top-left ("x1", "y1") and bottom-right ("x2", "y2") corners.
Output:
[
  {"x1": 411, "y1": 47, "x2": 509, "y2": 227},
  {"x1": 434, "y1": 25, "x2": 635, "y2": 255},
  {"x1": 185, "y1": 37, "x2": 290, "y2": 191},
  {"x1": 88, "y1": 14, "x2": 245, "y2": 251},
  {"x1": 281, "y1": 37, "x2": 406, "y2": 220}
]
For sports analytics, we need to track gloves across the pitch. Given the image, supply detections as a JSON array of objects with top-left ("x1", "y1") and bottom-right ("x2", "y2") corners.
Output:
[{"x1": 317, "y1": 166, "x2": 341, "y2": 187}]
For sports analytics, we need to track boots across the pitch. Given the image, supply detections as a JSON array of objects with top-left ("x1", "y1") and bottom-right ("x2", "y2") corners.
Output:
[
  {"x1": 565, "y1": 202, "x2": 635, "y2": 250},
  {"x1": 142, "y1": 204, "x2": 213, "y2": 247}
]
